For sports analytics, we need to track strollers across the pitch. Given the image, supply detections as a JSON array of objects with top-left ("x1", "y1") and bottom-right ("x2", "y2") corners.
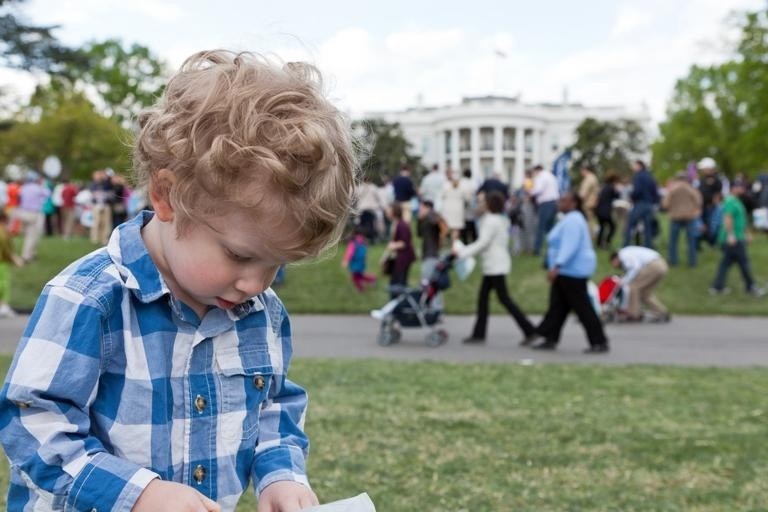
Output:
[{"x1": 370, "y1": 253, "x2": 457, "y2": 347}]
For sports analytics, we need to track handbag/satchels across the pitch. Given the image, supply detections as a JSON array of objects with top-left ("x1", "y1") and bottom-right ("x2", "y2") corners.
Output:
[{"x1": 378, "y1": 249, "x2": 399, "y2": 277}]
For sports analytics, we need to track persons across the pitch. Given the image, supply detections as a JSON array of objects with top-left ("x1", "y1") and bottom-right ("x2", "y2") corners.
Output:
[
  {"x1": 659, "y1": 170, "x2": 703, "y2": 267},
  {"x1": 450, "y1": 188, "x2": 536, "y2": 345},
  {"x1": 528, "y1": 165, "x2": 560, "y2": 255},
  {"x1": 621, "y1": 160, "x2": 659, "y2": 247},
  {"x1": 417, "y1": 200, "x2": 448, "y2": 260},
  {"x1": 0, "y1": 162, "x2": 135, "y2": 318},
  {"x1": 594, "y1": 171, "x2": 620, "y2": 247},
  {"x1": 353, "y1": 174, "x2": 391, "y2": 235},
  {"x1": 708, "y1": 192, "x2": 722, "y2": 242},
  {"x1": 470, "y1": 190, "x2": 486, "y2": 234},
  {"x1": 577, "y1": 166, "x2": 600, "y2": 238},
  {"x1": 342, "y1": 226, "x2": 375, "y2": 293},
  {"x1": 418, "y1": 164, "x2": 444, "y2": 204},
  {"x1": 434, "y1": 166, "x2": 471, "y2": 247},
  {"x1": 695, "y1": 157, "x2": 721, "y2": 248},
  {"x1": 610, "y1": 246, "x2": 670, "y2": 322},
  {"x1": 710, "y1": 178, "x2": 765, "y2": 296},
  {"x1": 0, "y1": 48, "x2": 363, "y2": 512},
  {"x1": 505, "y1": 188, "x2": 521, "y2": 253},
  {"x1": 479, "y1": 164, "x2": 507, "y2": 195},
  {"x1": 459, "y1": 168, "x2": 477, "y2": 244},
  {"x1": 393, "y1": 165, "x2": 416, "y2": 224},
  {"x1": 379, "y1": 203, "x2": 416, "y2": 300},
  {"x1": 530, "y1": 189, "x2": 607, "y2": 353}
]
